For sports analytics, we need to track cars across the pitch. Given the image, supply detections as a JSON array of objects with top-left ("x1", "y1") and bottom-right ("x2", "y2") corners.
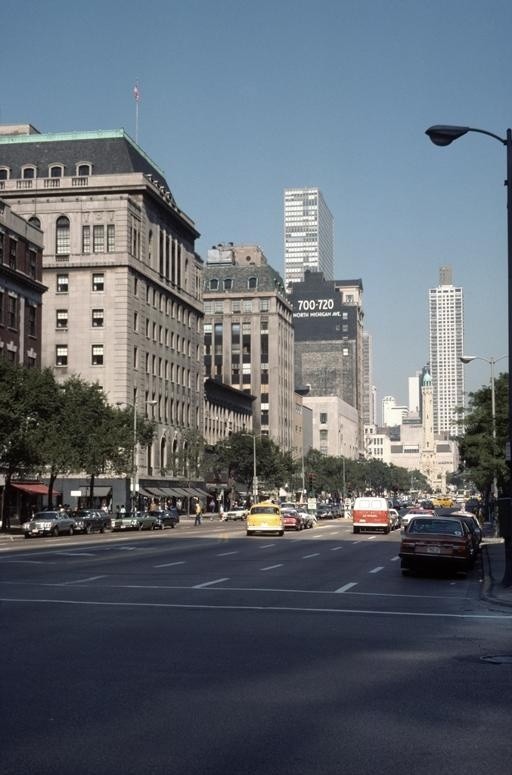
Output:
[
  {"x1": 19, "y1": 506, "x2": 180, "y2": 538},
  {"x1": 222, "y1": 492, "x2": 483, "y2": 577}
]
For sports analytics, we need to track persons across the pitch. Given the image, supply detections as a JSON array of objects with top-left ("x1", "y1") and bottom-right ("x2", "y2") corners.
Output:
[{"x1": 23, "y1": 495, "x2": 255, "y2": 526}]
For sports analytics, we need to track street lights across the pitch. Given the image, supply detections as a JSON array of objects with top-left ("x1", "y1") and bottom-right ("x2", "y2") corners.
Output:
[
  {"x1": 117, "y1": 400, "x2": 159, "y2": 512},
  {"x1": 461, "y1": 353, "x2": 509, "y2": 538},
  {"x1": 426, "y1": 124, "x2": 512, "y2": 374},
  {"x1": 240, "y1": 432, "x2": 268, "y2": 504}
]
